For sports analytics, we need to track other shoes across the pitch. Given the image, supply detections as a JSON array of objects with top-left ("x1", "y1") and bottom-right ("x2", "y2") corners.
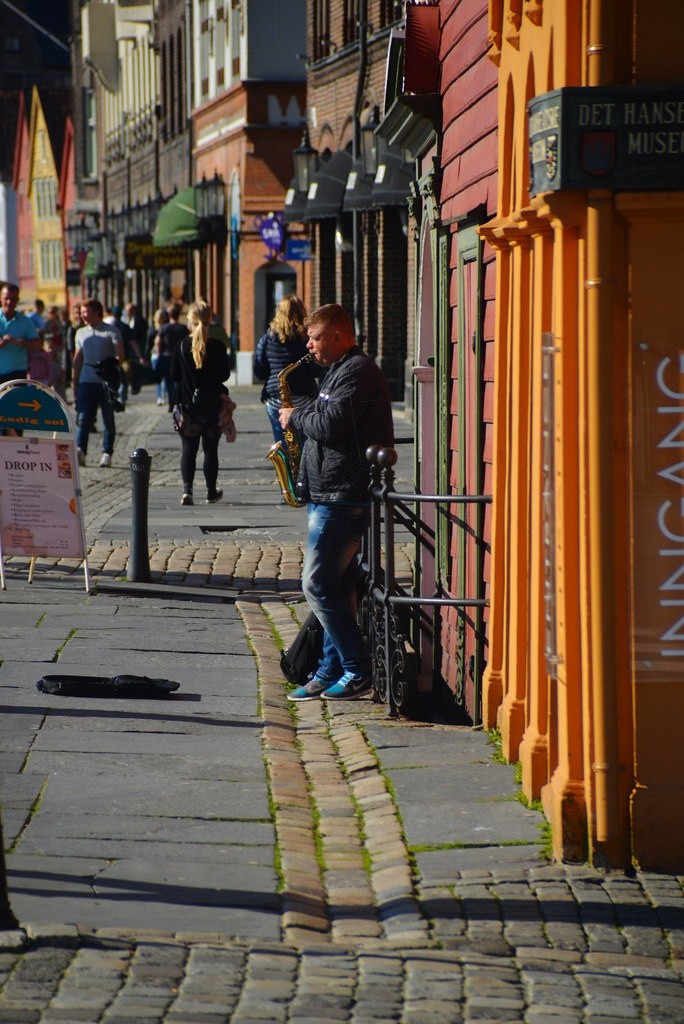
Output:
[
  {"x1": 100, "y1": 453, "x2": 111, "y2": 466},
  {"x1": 157, "y1": 398, "x2": 164, "y2": 404},
  {"x1": 77, "y1": 447, "x2": 85, "y2": 465}
]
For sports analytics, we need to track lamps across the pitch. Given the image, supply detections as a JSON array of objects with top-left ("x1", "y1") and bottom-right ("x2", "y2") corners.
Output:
[
  {"x1": 320, "y1": 38, "x2": 338, "y2": 54},
  {"x1": 296, "y1": 53, "x2": 310, "y2": 67},
  {"x1": 356, "y1": 20, "x2": 373, "y2": 34},
  {"x1": 361, "y1": 104, "x2": 388, "y2": 177},
  {"x1": 294, "y1": 122, "x2": 322, "y2": 195}
]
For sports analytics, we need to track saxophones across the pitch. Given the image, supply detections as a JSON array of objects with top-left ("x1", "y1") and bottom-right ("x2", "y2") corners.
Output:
[{"x1": 264, "y1": 349, "x2": 317, "y2": 510}]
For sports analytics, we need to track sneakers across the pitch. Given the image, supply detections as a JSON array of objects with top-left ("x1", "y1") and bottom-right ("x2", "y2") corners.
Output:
[
  {"x1": 320, "y1": 671, "x2": 372, "y2": 700},
  {"x1": 181, "y1": 493, "x2": 194, "y2": 506},
  {"x1": 287, "y1": 673, "x2": 339, "y2": 701},
  {"x1": 205, "y1": 488, "x2": 222, "y2": 503}
]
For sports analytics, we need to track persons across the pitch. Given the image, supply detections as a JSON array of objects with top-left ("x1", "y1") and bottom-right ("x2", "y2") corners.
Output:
[
  {"x1": 279, "y1": 302, "x2": 395, "y2": 703},
  {"x1": 1, "y1": 281, "x2": 233, "y2": 506},
  {"x1": 255, "y1": 295, "x2": 324, "y2": 506}
]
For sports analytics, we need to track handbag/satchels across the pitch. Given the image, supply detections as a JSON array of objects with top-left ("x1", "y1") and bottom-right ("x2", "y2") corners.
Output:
[{"x1": 170, "y1": 403, "x2": 192, "y2": 432}]
